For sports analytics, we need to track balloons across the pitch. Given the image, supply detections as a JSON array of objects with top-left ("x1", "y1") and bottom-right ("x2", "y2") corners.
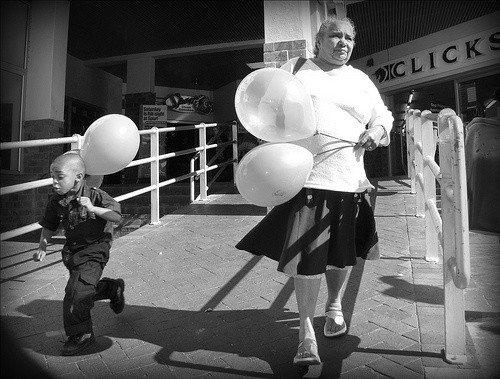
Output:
[
  {"x1": 234, "y1": 67, "x2": 319, "y2": 143},
  {"x1": 80, "y1": 114, "x2": 141, "y2": 176},
  {"x1": 234, "y1": 142, "x2": 314, "y2": 208}
]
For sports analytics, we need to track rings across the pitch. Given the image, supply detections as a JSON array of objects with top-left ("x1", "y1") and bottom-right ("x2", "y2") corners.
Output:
[{"x1": 368, "y1": 146, "x2": 373, "y2": 151}]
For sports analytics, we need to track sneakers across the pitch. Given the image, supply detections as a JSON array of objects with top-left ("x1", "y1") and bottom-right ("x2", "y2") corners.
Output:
[
  {"x1": 59, "y1": 332, "x2": 95, "y2": 355},
  {"x1": 109, "y1": 279, "x2": 125, "y2": 313}
]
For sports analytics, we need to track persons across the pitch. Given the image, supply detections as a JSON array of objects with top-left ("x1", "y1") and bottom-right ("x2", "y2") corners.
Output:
[
  {"x1": 257, "y1": 17, "x2": 394, "y2": 365},
  {"x1": 33, "y1": 151, "x2": 126, "y2": 355}
]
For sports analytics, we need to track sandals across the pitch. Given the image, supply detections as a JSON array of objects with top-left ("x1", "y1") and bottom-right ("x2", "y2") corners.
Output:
[
  {"x1": 293, "y1": 339, "x2": 321, "y2": 364},
  {"x1": 324, "y1": 310, "x2": 346, "y2": 336}
]
